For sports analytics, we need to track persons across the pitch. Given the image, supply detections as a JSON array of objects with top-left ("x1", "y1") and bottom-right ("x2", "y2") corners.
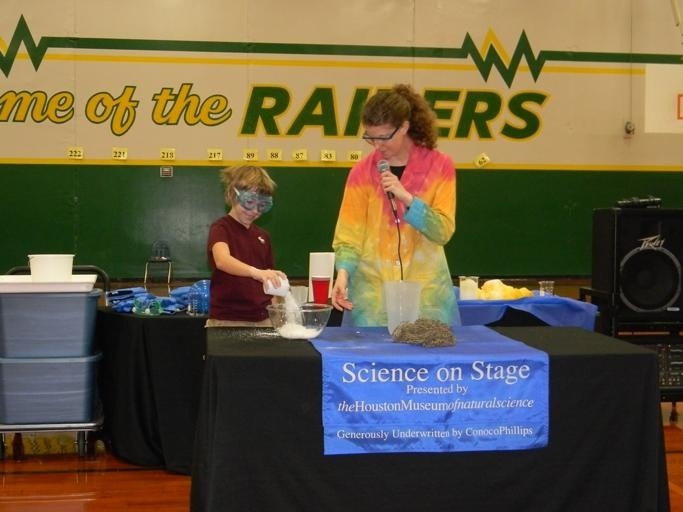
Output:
[
  {"x1": 207, "y1": 164, "x2": 285, "y2": 321},
  {"x1": 331, "y1": 84, "x2": 461, "y2": 327}
]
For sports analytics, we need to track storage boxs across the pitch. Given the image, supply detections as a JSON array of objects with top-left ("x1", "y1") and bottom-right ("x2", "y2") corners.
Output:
[
  {"x1": 1, "y1": 353, "x2": 103, "y2": 422},
  {"x1": 0, "y1": 289, "x2": 103, "y2": 358},
  {"x1": 29, "y1": 255, "x2": 74, "y2": 277}
]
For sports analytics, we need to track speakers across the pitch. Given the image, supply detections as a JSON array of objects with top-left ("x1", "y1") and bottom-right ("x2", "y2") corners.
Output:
[{"x1": 593, "y1": 207, "x2": 683, "y2": 324}]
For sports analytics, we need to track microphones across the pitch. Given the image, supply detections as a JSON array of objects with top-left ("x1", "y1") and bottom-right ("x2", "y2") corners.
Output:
[{"x1": 376, "y1": 158, "x2": 398, "y2": 212}]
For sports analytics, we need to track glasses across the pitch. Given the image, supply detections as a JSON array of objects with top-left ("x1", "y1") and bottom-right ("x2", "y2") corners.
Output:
[
  {"x1": 362, "y1": 125, "x2": 400, "y2": 145},
  {"x1": 232, "y1": 186, "x2": 274, "y2": 213}
]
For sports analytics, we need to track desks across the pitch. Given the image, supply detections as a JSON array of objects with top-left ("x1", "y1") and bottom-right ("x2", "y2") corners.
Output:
[
  {"x1": 327, "y1": 296, "x2": 344, "y2": 325},
  {"x1": 109, "y1": 309, "x2": 208, "y2": 471},
  {"x1": 453, "y1": 281, "x2": 599, "y2": 328},
  {"x1": 193, "y1": 326, "x2": 669, "y2": 512}
]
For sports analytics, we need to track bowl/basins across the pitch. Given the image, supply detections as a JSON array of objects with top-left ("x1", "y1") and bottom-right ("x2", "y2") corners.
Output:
[
  {"x1": 28, "y1": 254, "x2": 75, "y2": 281},
  {"x1": 266, "y1": 303, "x2": 334, "y2": 339}
]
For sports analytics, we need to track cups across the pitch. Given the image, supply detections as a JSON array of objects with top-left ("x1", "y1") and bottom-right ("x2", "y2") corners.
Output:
[
  {"x1": 312, "y1": 276, "x2": 330, "y2": 304},
  {"x1": 187, "y1": 292, "x2": 205, "y2": 317},
  {"x1": 538, "y1": 281, "x2": 555, "y2": 296},
  {"x1": 262, "y1": 275, "x2": 290, "y2": 297},
  {"x1": 383, "y1": 281, "x2": 422, "y2": 335},
  {"x1": 459, "y1": 276, "x2": 479, "y2": 300}
]
